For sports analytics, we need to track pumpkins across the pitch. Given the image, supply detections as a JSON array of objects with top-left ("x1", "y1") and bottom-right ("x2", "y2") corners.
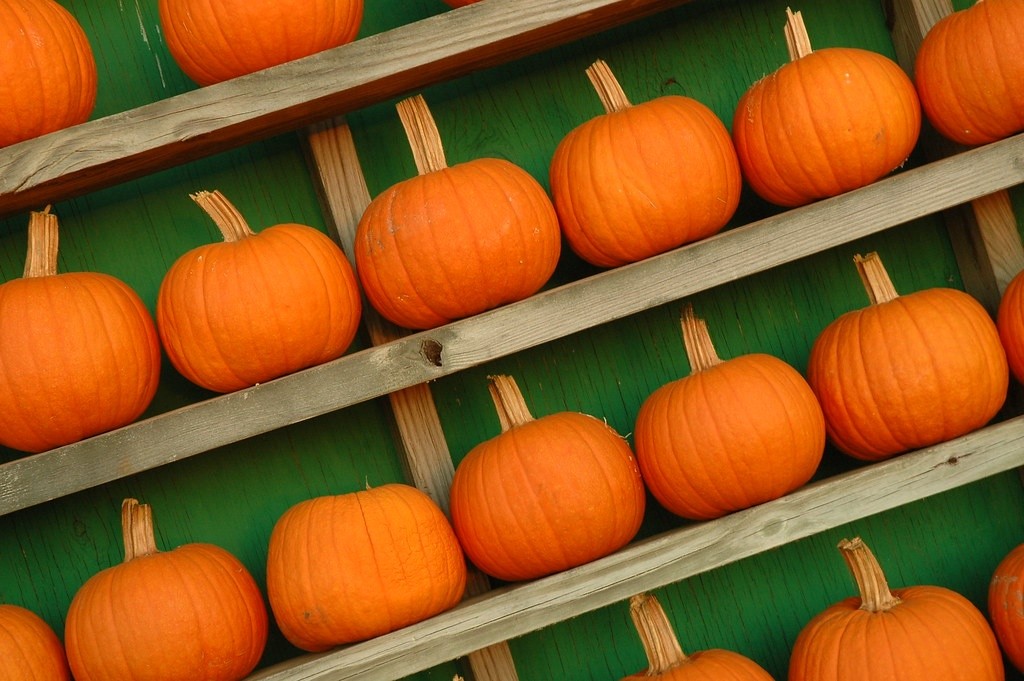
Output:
[{"x1": 0, "y1": 0, "x2": 1024, "y2": 681}]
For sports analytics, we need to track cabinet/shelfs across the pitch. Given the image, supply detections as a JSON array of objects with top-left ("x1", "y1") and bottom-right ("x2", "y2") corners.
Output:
[{"x1": 1, "y1": 1, "x2": 1023, "y2": 681}]
